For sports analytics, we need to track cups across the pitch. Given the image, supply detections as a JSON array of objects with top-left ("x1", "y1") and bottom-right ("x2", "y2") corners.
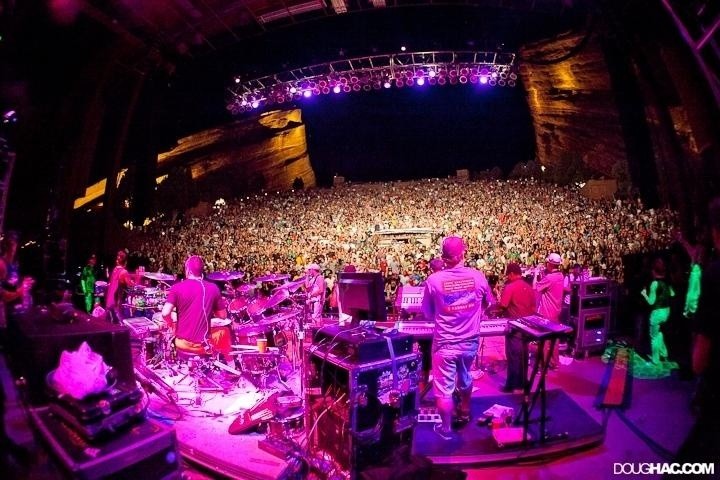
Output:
[{"x1": 256, "y1": 338, "x2": 268, "y2": 353}]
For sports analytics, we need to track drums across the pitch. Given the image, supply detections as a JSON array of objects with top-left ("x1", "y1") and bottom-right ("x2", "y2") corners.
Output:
[
  {"x1": 212, "y1": 296, "x2": 251, "y2": 319},
  {"x1": 210, "y1": 318, "x2": 234, "y2": 362},
  {"x1": 152, "y1": 312, "x2": 177, "y2": 333},
  {"x1": 241, "y1": 352, "x2": 281, "y2": 392},
  {"x1": 234, "y1": 324, "x2": 269, "y2": 350},
  {"x1": 247, "y1": 295, "x2": 272, "y2": 322}
]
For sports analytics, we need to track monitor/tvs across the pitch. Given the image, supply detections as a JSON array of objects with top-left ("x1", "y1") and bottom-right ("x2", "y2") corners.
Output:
[{"x1": 336, "y1": 270, "x2": 387, "y2": 335}]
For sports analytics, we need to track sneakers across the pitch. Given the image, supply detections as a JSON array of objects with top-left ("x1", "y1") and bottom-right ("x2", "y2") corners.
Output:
[
  {"x1": 503, "y1": 381, "x2": 532, "y2": 393},
  {"x1": 433, "y1": 415, "x2": 472, "y2": 441},
  {"x1": 542, "y1": 363, "x2": 561, "y2": 371}
]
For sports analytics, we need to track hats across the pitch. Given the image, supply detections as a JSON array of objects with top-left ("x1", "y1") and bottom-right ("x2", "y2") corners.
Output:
[
  {"x1": 506, "y1": 263, "x2": 522, "y2": 276},
  {"x1": 185, "y1": 255, "x2": 204, "y2": 277},
  {"x1": 307, "y1": 264, "x2": 320, "y2": 270},
  {"x1": 546, "y1": 253, "x2": 563, "y2": 265},
  {"x1": 441, "y1": 235, "x2": 468, "y2": 258}
]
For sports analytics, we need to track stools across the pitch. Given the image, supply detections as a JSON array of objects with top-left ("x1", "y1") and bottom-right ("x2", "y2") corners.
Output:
[{"x1": 176, "y1": 350, "x2": 207, "y2": 384}]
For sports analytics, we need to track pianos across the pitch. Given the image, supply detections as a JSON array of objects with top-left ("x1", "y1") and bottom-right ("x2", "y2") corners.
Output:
[
  {"x1": 508, "y1": 314, "x2": 573, "y2": 338},
  {"x1": 394, "y1": 287, "x2": 426, "y2": 310},
  {"x1": 395, "y1": 318, "x2": 510, "y2": 339}
]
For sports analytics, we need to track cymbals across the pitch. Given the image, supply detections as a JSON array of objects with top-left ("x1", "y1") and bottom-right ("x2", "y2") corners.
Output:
[
  {"x1": 206, "y1": 271, "x2": 245, "y2": 280},
  {"x1": 265, "y1": 278, "x2": 307, "y2": 309},
  {"x1": 237, "y1": 284, "x2": 257, "y2": 292},
  {"x1": 253, "y1": 274, "x2": 291, "y2": 281},
  {"x1": 258, "y1": 312, "x2": 299, "y2": 325},
  {"x1": 143, "y1": 272, "x2": 175, "y2": 281}
]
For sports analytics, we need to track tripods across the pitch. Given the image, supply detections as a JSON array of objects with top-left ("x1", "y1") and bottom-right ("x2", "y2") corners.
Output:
[{"x1": 130, "y1": 294, "x2": 311, "y2": 408}]
